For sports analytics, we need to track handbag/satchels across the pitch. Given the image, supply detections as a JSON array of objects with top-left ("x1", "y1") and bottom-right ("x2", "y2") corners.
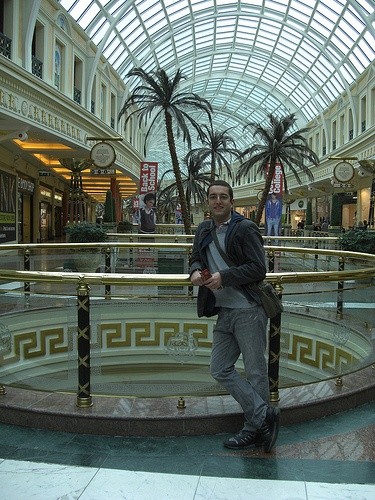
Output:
[{"x1": 253, "y1": 282, "x2": 284, "y2": 318}]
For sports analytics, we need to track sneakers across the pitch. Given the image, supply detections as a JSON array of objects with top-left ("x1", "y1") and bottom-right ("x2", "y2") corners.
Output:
[
  {"x1": 261, "y1": 406, "x2": 280, "y2": 452},
  {"x1": 223, "y1": 429, "x2": 264, "y2": 449}
]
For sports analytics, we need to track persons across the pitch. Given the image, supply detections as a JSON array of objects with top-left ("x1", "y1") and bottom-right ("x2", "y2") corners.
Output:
[
  {"x1": 313, "y1": 220, "x2": 329, "y2": 237},
  {"x1": 188, "y1": 180, "x2": 281, "y2": 453},
  {"x1": 266, "y1": 192, "x2": 282, "y2": 245},
  {"x1": 139, "y1": 192, "x2": 157, "y2": 243},
  {"x1": 297, "y1": 220, "x2": 305, "y2": 229}
]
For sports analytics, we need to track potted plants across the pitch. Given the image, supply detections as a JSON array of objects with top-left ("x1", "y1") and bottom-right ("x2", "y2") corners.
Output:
[
  {"x1": 117, "y1": 220, "x2": 133, "y2": 241},
  {"x1": 335, "y1": 228, "x2": 375, "y2": 283},
  {"x1": 64, "y1": 222, "x2": 108, "y2": 273}
]
